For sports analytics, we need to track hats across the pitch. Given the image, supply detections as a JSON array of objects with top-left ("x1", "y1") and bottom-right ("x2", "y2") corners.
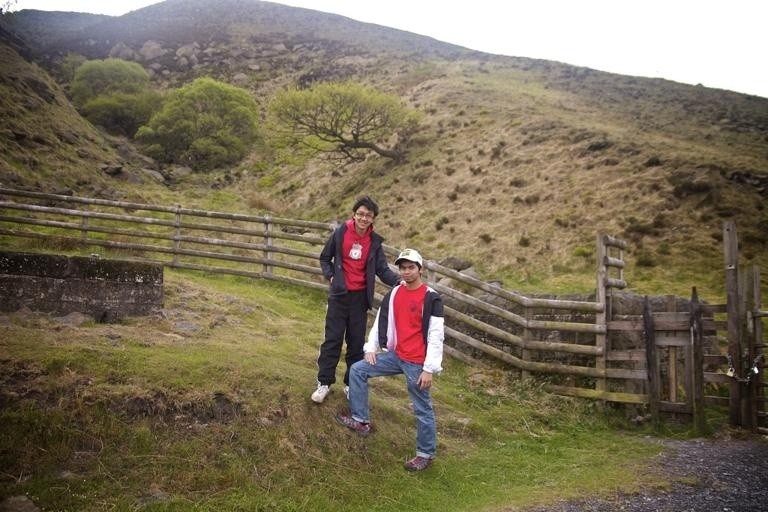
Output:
[{"x1": 394, "y1": 248, "x2": 422, "y2": 267}]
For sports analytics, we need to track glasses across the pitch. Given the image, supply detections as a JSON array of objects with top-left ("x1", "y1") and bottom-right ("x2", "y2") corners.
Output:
[{"x1": 354, "y1": 211, "x2": 373, "y2": 219}]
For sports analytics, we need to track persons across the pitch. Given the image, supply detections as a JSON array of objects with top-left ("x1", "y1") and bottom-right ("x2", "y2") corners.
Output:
[
  {"x1": 336, "y1": 249, "x2": 445, "y2": 471},
  {"x1": 311, "y1": 195, "x2": 405, "y2": 405}
]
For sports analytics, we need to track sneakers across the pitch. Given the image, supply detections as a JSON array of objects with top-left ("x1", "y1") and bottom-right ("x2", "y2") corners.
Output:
[
  {"x1": 335, "y1": 411, "x2": 369, "y2": 433},
  {"x1": 343, "y1": 385, "x2": 350, "y2": 400},
  {"x1": 311, "y1": 381, "x2": 330, "y2": 403},
  {"x1": 403, "y1": 456, "x2": 430, "y2": 471}
]
